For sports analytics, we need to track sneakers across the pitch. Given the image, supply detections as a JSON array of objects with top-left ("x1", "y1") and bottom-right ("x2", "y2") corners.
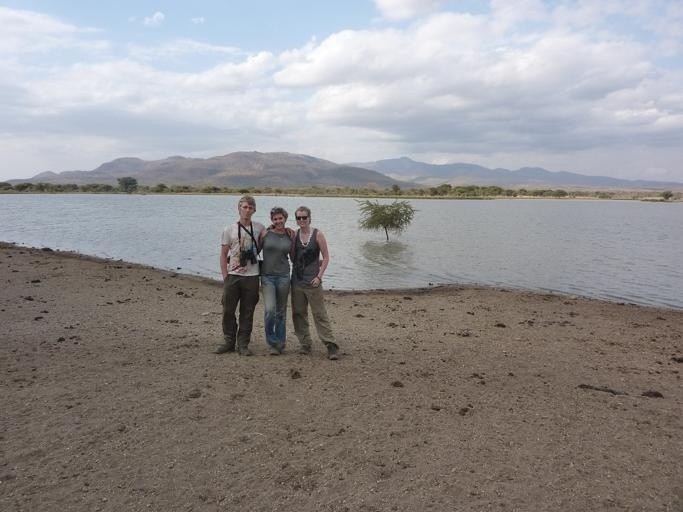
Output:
[
  {"x1": 328, "y1": 343, "x2": 338, "y2": 360},
  {"x1": 214, "y1": 342, "x2": 235, "y2": 353},
  {"x1": 237, "y1": 346, "x2": 252, "y2": 356},
  {"x1": 267, "y1": 335, "x2": 284, "y2": 355},
  {"x1": 299, "y1": 344, "x2": 311, "y2": 354}
]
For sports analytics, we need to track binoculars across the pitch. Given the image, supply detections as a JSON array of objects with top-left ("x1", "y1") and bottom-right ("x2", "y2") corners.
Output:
[{"x1": 240, "y1": 250, "x2": 257, "y2": 267}]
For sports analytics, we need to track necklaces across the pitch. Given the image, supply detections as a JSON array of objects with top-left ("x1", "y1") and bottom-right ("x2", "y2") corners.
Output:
[
  {"x1": 273, "y1": 226, "x2": 285, "y2": 240},
  {"x1": 300, "y1": 227, "x2": 313, "y2": 247}
]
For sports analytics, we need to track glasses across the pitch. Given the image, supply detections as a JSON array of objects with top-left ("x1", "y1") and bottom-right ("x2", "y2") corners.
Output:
[{"x1": 296, "y1": 216, "x2": 308, "y2": 220}]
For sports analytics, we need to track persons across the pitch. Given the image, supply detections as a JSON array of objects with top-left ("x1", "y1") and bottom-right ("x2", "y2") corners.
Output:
[
  {"x1": 224, "y1": 207, "x2": 324, "y2": 356},
  {"x1": 265, "y1": 205, "x2": 339, "y2": 360},
  {"x1": 213, "y1": 196, "x2": 295, "y2": 356}
]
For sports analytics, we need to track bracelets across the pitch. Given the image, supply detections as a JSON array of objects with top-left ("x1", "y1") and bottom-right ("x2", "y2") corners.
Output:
[{"x1": 317, "y1": 275, "x2": 321, "y2": 281}]
